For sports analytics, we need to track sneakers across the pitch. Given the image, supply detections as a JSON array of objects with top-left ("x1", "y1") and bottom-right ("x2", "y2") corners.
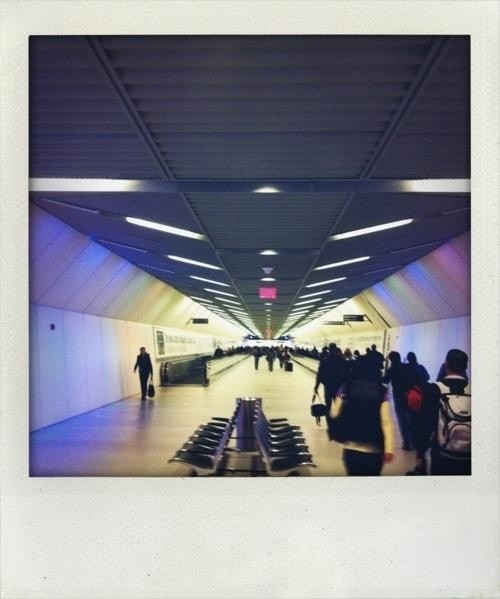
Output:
[{"x1": 406, "y1": 467, "x2": 426, "y2": 475}]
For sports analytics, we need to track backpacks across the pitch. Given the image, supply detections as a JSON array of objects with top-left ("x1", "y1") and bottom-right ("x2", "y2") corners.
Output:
[{"x1": 433, "y1": 382, "x2": 471, "y2": 464}]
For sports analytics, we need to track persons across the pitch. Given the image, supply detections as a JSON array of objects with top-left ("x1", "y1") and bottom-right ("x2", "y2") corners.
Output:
[
  {"x1": 228, "y1": 342, "x2": 387, "y2": 377},
  {"x1": 416, "y1": 348, "x2": 471, "y2": 475},
  {"x1": 133, "y1": 346, "x2": 154, "y2": 400},
  {"x1": 384, "y1": 349, "x2": 413, "y2": 452},
  {"x1": 214, "y1": 345, "x2": 224, "y2": 357},
  {"x1": 407, "y1": 351, "x2": 431, "y2": 382},
  {"x1": 312, "y1": 342, "x2": 348, "y2": 424},
  {"x1": 328, "y1": 351, "x2": 396, "y2": 476}
]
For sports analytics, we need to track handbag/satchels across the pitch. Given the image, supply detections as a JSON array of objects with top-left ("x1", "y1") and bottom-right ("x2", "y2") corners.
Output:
[
  {"x1": 148, "y1": 384, "x2": 155, "y2": 397},
  {"x1": 311, "y1": 404, "x2": 326, "y2": 417},
  {"x1": 327, "y1": 407, "x2": 359, "y2": 441}
]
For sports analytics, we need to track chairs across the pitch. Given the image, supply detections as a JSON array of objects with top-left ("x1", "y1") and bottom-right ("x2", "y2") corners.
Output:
[{"x1": 168, "y1": 397, "x2": 317, "y2": 477}]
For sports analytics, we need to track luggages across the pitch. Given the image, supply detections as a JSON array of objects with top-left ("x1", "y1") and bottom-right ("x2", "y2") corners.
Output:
[{"x1": 286, "y1": 363, "x2": 292, "y2": 371}]
